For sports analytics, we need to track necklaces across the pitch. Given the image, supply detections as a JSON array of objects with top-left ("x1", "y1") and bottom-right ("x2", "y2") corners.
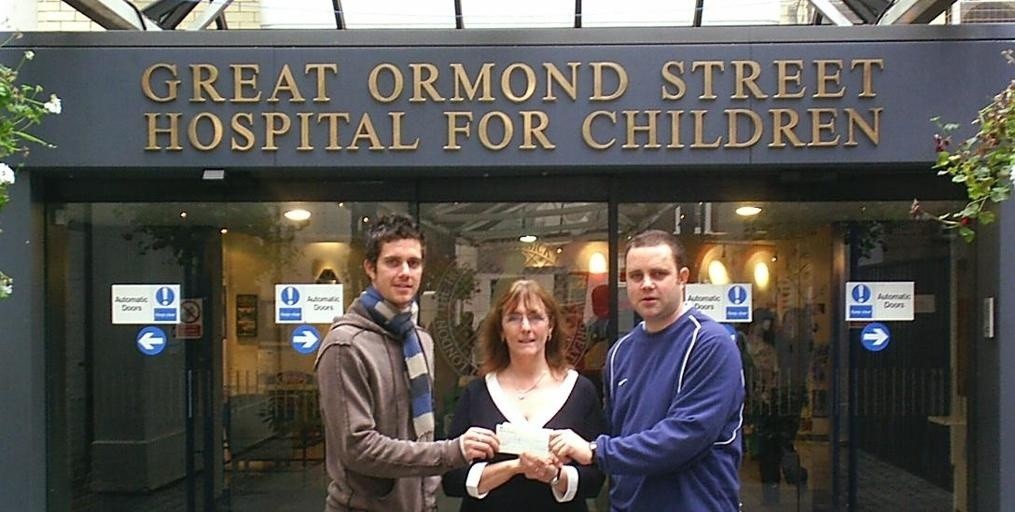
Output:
[{"x1": 508, "y1": 371, "x2": 546, "y2": 400}]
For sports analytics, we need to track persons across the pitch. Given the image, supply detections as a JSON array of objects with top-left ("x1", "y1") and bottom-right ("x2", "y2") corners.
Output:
[
  {"x1": 455, "y1": 311, "x2": 477, "y2": 357},
  {"x1": 547, "y1": 230, "x2": 745, "y2": 512},
  {"x1": 734, "y1": 307, "x2": 814, "y2": 510},
  {"x1": 312, "y1": 213, "x2": 501, "y2": 512},
  {"x1": 470, "y1": 319, "x2": 495, "y2": 371},
  {"x1": 587, "y1": 285, "x2": 612, "y2": 347},
  {"x1": 439, "y1": 280, "x2": 610, "y2": 512}
]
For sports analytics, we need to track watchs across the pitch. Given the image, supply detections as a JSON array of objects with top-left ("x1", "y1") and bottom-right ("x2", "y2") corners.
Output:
[
  {"x1": 547, "y1": 468, "x2": 561, "y2": 487},
  {"x1": 590, "y1": 440, "x2": 598, "y2": 464}
]
